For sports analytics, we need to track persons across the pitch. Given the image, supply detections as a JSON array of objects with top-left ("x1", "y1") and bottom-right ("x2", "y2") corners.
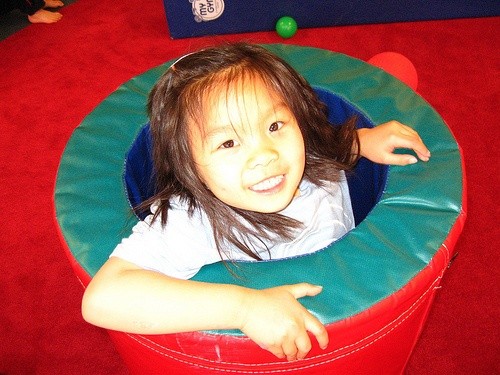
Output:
[
  {"x1": 0, "y1": 0, "x2": 64, "y2": 23},
  {"x1": 81, "y1": 41, "x2": 431, "y2": 361}
]
